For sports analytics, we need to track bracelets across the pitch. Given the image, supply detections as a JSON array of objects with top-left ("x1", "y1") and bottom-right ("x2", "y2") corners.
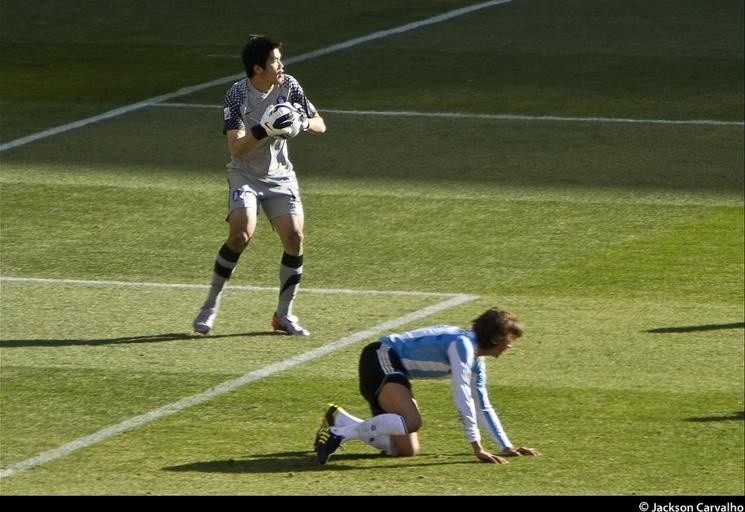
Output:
[{"x1": 251, "y1": 125, "x2": 267, "y2": 140}]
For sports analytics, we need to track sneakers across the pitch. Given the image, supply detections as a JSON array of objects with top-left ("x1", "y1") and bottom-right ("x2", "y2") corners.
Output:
[
  {"x1": 193, "y1": 306, "x2": 219, "y2": 334},
  {"x1": 313, "y1": 403, "x2": 343, "y2": 452},
  {"x1": 317, "y1": 425, "x2": 342, "y2": 464},
  {"x1": 272, "y1": 311, "x2": 311, "y2": 337}
]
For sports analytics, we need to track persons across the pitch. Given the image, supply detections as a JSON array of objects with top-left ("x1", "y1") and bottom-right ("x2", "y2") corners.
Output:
[
  {"x1": 316, "y1": 306, "x2": 542, "y2": 466},
  {"x1": 193, "y1": 37, "x2": 326, "y2": 337}
]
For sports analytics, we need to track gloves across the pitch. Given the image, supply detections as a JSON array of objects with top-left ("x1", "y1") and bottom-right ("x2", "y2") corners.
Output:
[
  {"x1": 292, "y1": 102, "x2": 310, "y2": 133},
  {"x1": 251, "y1": 104, "x2": 296, "y2": 141}
]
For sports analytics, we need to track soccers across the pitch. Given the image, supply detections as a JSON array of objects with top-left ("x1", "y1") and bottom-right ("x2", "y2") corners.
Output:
[{"x1": 269, "y1": 101, "x2": 301, "y2": 138}]
